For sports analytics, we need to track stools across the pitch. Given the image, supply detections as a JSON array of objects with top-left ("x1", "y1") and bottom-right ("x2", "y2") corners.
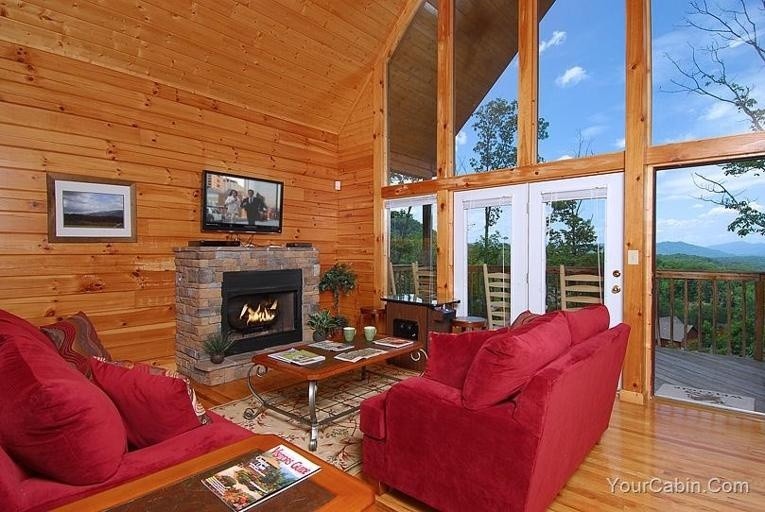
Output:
[
  {"x1": 451, "y1": 317, "x2": 488, "y2": 334},
  {"x1": 360, "y1": 307, "x2": 386, "y2": 332}
]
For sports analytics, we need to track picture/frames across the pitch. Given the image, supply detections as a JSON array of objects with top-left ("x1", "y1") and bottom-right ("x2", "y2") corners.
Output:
[{"x1": 46, "y1": 170, "x2": 137, "y2": 243}]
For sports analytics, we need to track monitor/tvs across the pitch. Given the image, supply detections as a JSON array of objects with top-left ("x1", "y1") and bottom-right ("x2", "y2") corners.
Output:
[{"x1": 202, "y1": 170, "x2": 283, "y2": 234}]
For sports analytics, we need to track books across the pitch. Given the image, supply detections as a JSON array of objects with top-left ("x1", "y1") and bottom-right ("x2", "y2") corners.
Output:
[
  {"x1": 373, "y1": 337, "x2": 414, "y2": 348},
  {"x1": 307, "y1": 340, "x2": 355, "y2": 352},
  {"x1": 267, "y1": 347, "x2": 325, "y2": 365},
  {"x1": 201, "y1": 445, "x2": 322, "y2": 512},
  {"x1": 334, "y1": 348, "x2": 388, "y2": 363}
]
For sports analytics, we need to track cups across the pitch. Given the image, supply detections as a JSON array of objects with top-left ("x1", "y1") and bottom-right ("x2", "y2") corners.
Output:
[
  {"x1": 343, "y1": 327, "x2": 356, "y2": 342},
  {"x1": 364, "y1": 326, "x2": 377, "y2": 341}
]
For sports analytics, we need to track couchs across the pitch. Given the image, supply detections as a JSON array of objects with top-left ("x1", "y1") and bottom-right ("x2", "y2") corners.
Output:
[
  {"x1": 0, "y1": 311, "x2": 254, "y2": 511},
  {"x1": 359, "y1": 304, "x2": 631, "y2": 512}
]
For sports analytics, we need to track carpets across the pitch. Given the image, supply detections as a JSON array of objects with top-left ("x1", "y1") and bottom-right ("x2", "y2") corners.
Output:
[{"x1": 209, "y1": 365, "x2": 424, "y2": 476}]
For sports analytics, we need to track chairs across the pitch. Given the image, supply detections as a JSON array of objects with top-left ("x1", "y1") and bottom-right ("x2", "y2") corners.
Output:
[
  {"x1": 483, "y1": 263, "x2": 511, "y2": 330},
  {"x1": 411, "y1": 263, "x2": 436, "y2": 302},
  {"x1": 388, "y1": 261, "x2": 420, "y2": 298},
  {"x1": 560, "y1": 263, "x2": 604, "y2": 310}
]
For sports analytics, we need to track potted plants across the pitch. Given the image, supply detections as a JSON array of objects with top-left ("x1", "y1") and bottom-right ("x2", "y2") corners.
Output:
[
  {"x1": 320, "y1": 262, "x2": 359, "y2": 340},
  {"x1": 307, "y1": 310, "x2": 336, "y2": 341},
  {"x1": 201, "y1": 333, "x2": 234, "y2": 363}
]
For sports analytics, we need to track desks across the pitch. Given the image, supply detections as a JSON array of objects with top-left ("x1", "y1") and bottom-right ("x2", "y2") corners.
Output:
[
  {"x1": 51, "y1": 436, "x2": 376, "y2": 512},
  {"x1": 244, "y1": 333, "x2": 428, "y2": 451}
]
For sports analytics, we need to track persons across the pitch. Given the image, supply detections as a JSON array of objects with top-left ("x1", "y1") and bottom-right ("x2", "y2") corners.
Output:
[
  {"x1": 240, "y1": 189, "x2": 260, "y2": 225},
  {"x1": 225, "y1": 190, "x2": 241, "y2": 224}
]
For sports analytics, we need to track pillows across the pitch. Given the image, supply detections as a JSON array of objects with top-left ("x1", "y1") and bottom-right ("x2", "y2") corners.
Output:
[
  {"x1": 429, "y1": 329, "x2": 509, "y2": 388},
  {"x1": 40, "y1": 311, "x2": 112, "y2": 381},
  {"x1": 564, "y1": 304, "x2": 610, "y2": 343},
  {"x1": 88, "y1": 353, "x2": 213, "y2": 449},
  {"x1": 1, "y1": 310, "x2": 127, "y2": 486},
  {"x1": 463, "y1": 311, "x2": 571, "y2": 411}
]
